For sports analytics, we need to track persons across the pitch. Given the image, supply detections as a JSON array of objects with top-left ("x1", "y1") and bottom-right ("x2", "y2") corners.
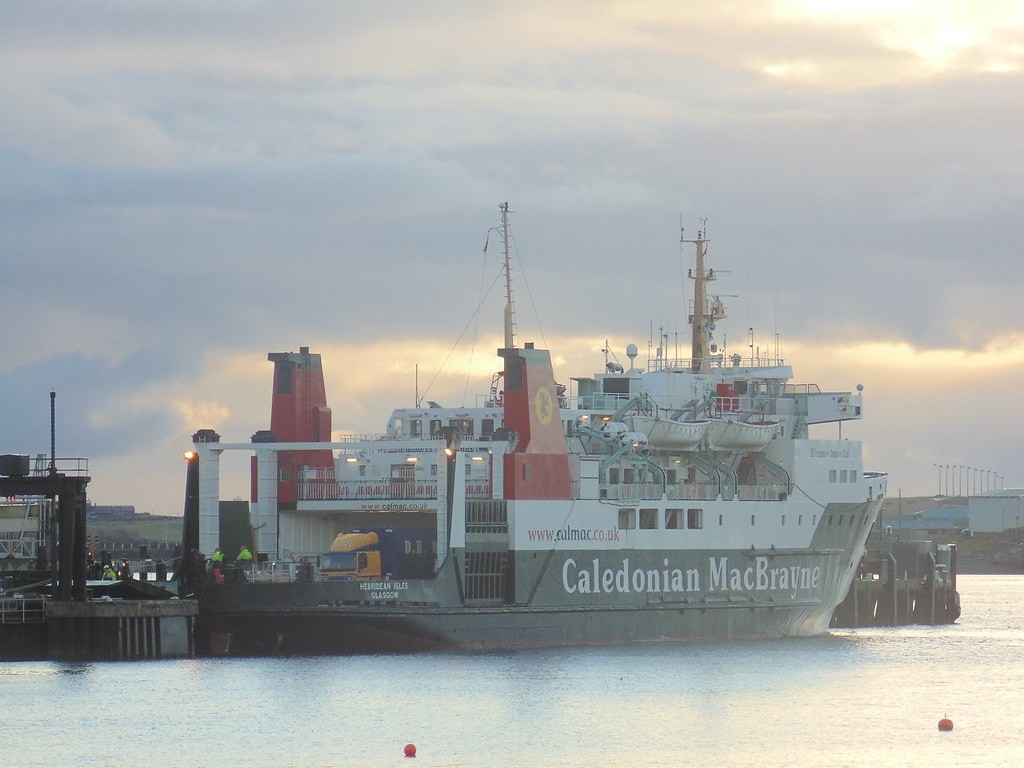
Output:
[
  {"x1": 211, "y1": 547, "x2": 224, "y2": 569},
  {"x1": 120, "y1": 558, "x2": 130, "y2": 574},
  {"x1": 102, "y1": 565, "x2": 116, "y2": 581},
  {"x1": 234, "y1": 546, "x2": 253, "y2": 563}
]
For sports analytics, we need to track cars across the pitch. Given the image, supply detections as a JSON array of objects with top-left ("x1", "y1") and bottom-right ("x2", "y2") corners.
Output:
[{"x1": 960, "y1": 529, "x2": 973, "y2": 538}]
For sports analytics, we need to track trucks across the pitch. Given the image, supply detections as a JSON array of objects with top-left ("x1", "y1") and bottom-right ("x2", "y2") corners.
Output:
[{"x1": 316, "y1": 527, "x2": 437, "y2": 581}]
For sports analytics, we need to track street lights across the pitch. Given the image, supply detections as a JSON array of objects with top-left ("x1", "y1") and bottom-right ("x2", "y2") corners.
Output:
[
  {"x1": 959, "y1": 466, "x2": 964, "y2": 497},
  {"x1": 938, "y1": 464, "x2": 943, "y2": 495},
  {"x1": 966, "y1": 467, "x2": 971, "y2": 496},
  {"x1": 945, "y1": 464, "x2": 950, "y2": 496},
  {"x1": 952, "y1": 465, "x2": 956, "y2": 497},
  {"x1": 974, "y1": 468, "x2": 978, "y2": 495},
  {"x1": 980, "y1": 470, "x2": 984, "y2": 494},
  {"x1": 899, "y1": 489, "x2": 901, "y2": 536},
  {"x1": 994, "y1": 472, "x2": 1004, "y2": 490},
  {"x1": 987, "y1": 471, "x2": 991, "y2": 491}
]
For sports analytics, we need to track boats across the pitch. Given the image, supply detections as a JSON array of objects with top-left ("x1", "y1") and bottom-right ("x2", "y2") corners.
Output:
[
  {"x1": 707, "y1": 417, "x2": 782, "y2": 452},
  {"x1": 182, "y1": 201, "x2": 887, "y2": 660},
  {"x1": 621, "y1": 411, "x2": 711, "y2": 452}
]
